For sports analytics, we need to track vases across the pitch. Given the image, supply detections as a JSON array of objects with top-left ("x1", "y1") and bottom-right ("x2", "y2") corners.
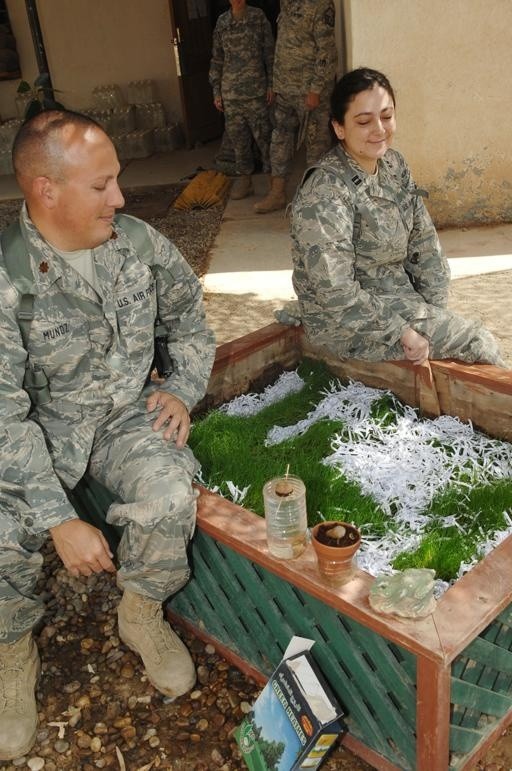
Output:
[{"x1": 311, "y1": 520, "x2": 361, "y2": 578}]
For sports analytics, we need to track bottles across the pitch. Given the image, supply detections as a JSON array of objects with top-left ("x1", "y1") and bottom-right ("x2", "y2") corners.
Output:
[
  {"x1": 75, "y1": 79, "x2": 184, "y2": 161},
  {"x1": 0, "y1": 118, "x2": 25, "y2": 177},
  {"x1": 262, "y1": 478, "x2": 309, "y2": 559}
]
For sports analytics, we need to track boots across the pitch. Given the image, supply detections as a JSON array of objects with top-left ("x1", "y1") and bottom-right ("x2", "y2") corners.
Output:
[
  {"x1": 265, "y1": 174, "x2": 273, "y2": 190},
  {"x1": 254, "y1": 178, "x2": 287, "y2": 213},
  {"x1": 118, "y1": 589, "x2": 197, "y2": 698},
  {"x1": 0, "y1": 631, "x2": 41, "y2": 760},
  {"x1": 231, "y1": 174, "x2": 255, "y2": 200}
]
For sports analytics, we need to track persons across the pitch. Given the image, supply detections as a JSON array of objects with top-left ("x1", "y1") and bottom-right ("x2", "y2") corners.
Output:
[
  {"x1": 273, "y1": 67, "x2": 512, "y2": 371},
  {"x1": 208, "y1": 0, "x2": 275, "y2": 200},
  {"x1": 252, "y1": 0, "x2": 339, "y2": 214},
  {"x1": 0, "y1": 107, "x2": 218, "y2": 761}
]
